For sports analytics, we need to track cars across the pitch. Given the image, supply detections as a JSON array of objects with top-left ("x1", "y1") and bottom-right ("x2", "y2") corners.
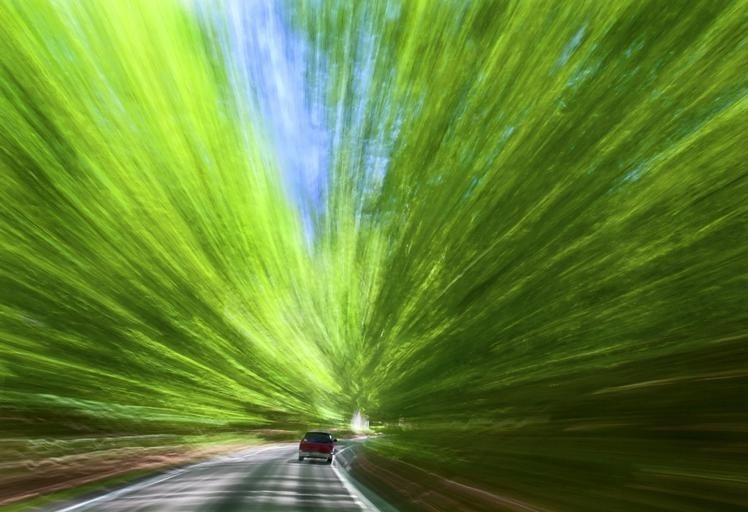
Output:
[{"x1": 295, "y1": 426, "x2": 337, "y2": 466}]
